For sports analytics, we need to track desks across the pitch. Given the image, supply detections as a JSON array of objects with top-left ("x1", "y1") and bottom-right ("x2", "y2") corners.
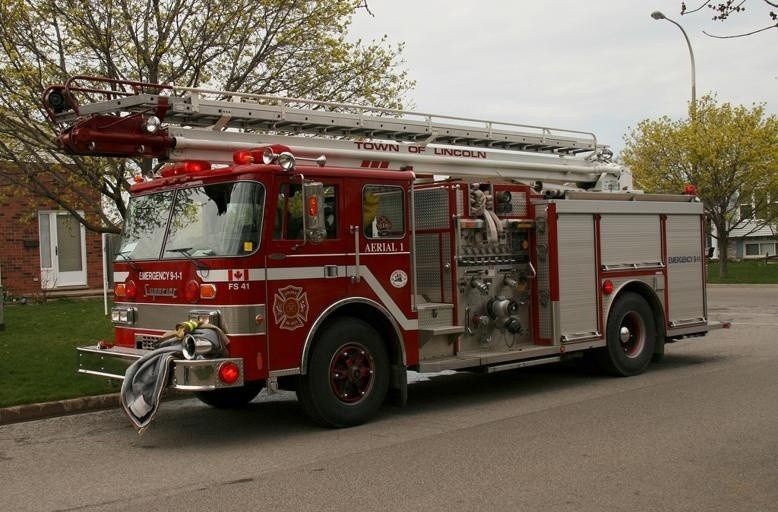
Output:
[{"x1": 652, "y1": 11, "x2": 697, "y2": 184}]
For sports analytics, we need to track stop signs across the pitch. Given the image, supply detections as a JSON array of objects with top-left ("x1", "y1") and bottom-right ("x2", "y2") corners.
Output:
[{"x1": 39, "y1": 76, "x2": 732, "y2": 431}]
